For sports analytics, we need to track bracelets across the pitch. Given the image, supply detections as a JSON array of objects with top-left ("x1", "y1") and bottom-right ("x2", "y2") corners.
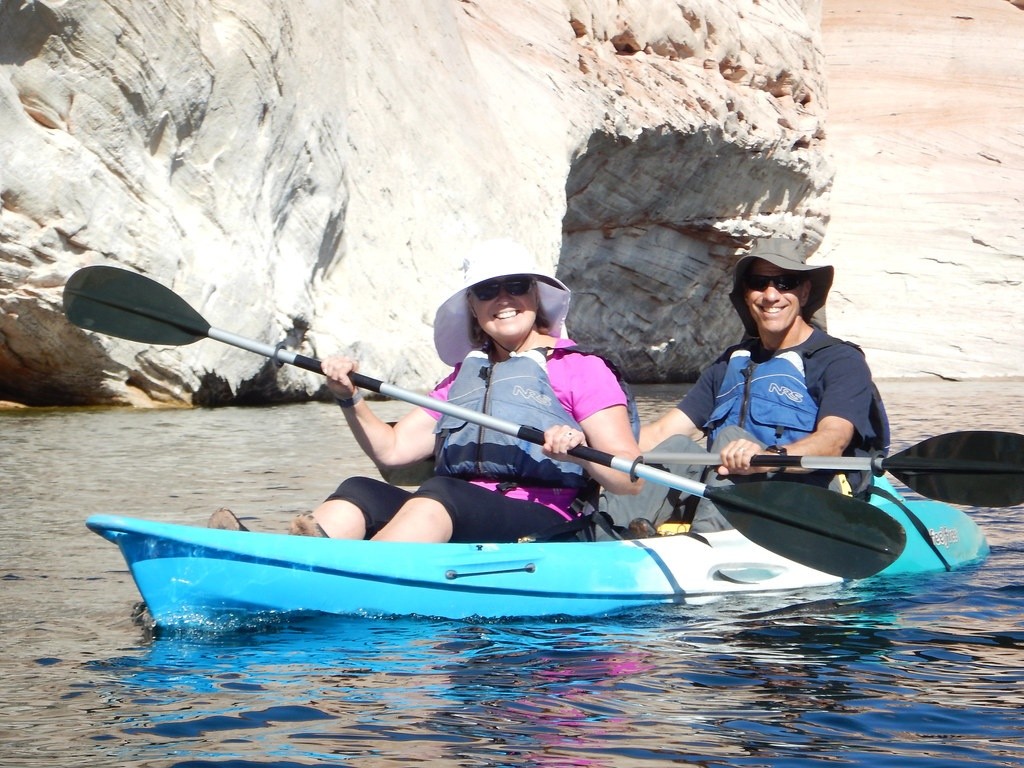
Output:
[{"x1": 335, "y1": 387, "x2": 362, "y2": 407}]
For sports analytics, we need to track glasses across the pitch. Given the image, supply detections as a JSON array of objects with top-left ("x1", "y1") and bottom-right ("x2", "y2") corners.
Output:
[
  {"x1": 744, "y1": 271, "x2": 812, "y2": 291},
  {"x1": 467, "y1": 275, "x2": 535, "y2": 300}
]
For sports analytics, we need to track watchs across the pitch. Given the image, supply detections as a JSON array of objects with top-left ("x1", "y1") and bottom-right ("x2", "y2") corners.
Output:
[{"x1": 765, "y1": 444, "x2": 788, "y2": 474}]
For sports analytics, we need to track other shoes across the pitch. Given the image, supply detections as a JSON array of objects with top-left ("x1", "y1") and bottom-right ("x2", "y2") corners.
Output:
[
  {"x1": 208, "y1": 508, "x2": 251, "y2": 531},
  {"x1": 290, "y1": 513, "x2": 329, "y2": 538},
  {"x1": 629, "y1": 517, "x2": 664, "y2": 539}
]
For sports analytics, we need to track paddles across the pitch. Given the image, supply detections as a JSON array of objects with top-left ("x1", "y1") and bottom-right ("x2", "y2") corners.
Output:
[
  {"x1": 374, "y1": 415, "x2": 1024, "y2": 509},
  {"x1": 60, "y1": 261, "x2": 910, "y2": 580}
]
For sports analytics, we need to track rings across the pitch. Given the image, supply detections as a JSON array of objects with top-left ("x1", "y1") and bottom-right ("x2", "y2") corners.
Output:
[
  {"x1": 737, "y1": 447, "x2": 744, "y2": 452},
  {"x1": 565, "y1": 431, "x2": 575, "y2": 439}
]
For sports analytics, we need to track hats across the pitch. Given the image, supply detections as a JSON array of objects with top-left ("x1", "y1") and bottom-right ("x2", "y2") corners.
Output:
[
  {"x1": 728, "y1": 238, "x2": 834, "y2": 337},
  {"x1": 433, "y1": 239, "x2": 571, "y2": 368}
]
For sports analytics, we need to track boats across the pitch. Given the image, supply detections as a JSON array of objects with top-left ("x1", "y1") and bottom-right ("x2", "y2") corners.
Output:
[{"x1": 84, "y1": 468, "x2": 992, "y2": 631}]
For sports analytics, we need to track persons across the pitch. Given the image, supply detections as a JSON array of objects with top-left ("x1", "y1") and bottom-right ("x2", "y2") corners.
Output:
[
  {"x1": 576, "y1": 236, "x2": 891, "y2": 543},
  {"x1": 208, "y1": 236, "x2": 644, "y2": 543}
]
